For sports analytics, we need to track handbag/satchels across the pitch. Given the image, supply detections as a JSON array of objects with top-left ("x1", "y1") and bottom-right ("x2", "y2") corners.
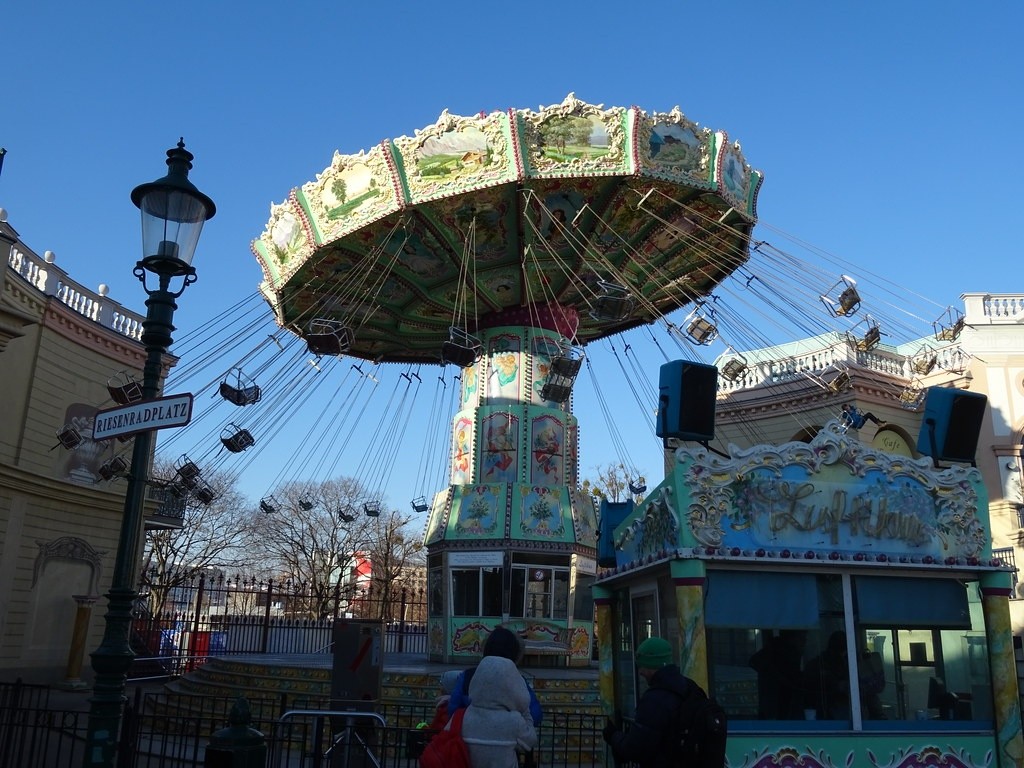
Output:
[{"x1": 858, "y1": 647, "x2": 886, "y2": 699}]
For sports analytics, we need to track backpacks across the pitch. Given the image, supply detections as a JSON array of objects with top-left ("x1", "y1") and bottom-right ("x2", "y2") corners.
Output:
[
  {"x1": 661, "y1": 681, "x2": 727, "y2": 768},
  {"x1": 418, "y1": 708, "x2": 473, "y2": 768}
]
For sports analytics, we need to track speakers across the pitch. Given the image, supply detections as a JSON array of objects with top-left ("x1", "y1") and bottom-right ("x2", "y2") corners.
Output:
[
  {"x1": 656, "y1": 359, "x2": 718, "y2": 440},
  {"x1": 917, "y1": 387, "x2": 987, "y2": 463}
]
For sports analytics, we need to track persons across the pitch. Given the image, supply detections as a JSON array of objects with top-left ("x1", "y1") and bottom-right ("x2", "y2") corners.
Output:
[
  {"x1": 842, "y1": 403, "x2": 887, "y2": 430},
  {"x1": 203, "y1": 697, "x2": 267, "y2": 768},
  {"x1": 602, "y1": 637, "x2": 707, "y2": 768},
  {"x1": 802, "y1": 629, "x2": 847, "y2": 721},
  {"x1": 440, "y1": 627, "x2": 543, "y2": 768}
]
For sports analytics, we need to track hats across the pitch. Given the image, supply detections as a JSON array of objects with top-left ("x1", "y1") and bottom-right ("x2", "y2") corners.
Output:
[{"x1": 636, "y1": 636, "x2": 676, "y2": 669}]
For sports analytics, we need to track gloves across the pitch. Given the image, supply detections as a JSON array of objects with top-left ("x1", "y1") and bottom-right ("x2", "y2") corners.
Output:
[{"x1": 602, "y1": 720, "x2": 615, "y2": 745}]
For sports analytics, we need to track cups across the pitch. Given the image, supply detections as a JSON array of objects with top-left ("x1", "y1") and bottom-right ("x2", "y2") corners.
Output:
[
  {"x1": 916, "y1": 709, "x2": 928, "y2": 720},
  {"x1": 805, "y1": 710, "x2": 816, "y2": 721}
]
